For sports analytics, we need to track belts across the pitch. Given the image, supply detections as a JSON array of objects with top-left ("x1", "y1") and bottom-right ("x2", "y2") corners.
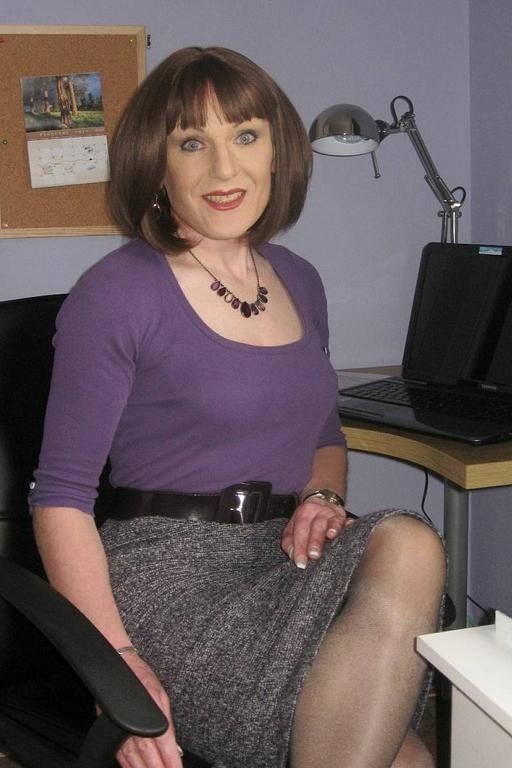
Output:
[{"x1": 110, "y1": 487, "x2": 299, "y2": 525}]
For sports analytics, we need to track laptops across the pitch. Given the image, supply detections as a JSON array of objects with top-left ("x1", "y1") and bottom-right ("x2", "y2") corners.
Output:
[{"x1": 336, "y1": 242, "x2": 512, "y2": 445}]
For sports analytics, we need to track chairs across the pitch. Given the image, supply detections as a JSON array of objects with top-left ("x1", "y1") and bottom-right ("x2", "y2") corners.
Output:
[{"x1": 1, "y1": 293, "x2": 456, "y2": 768}]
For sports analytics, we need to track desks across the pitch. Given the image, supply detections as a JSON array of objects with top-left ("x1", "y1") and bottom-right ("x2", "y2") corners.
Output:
[
  {"x1": 416, "y1": 624, "x2": 512, "y2": 768},
  {"x1": 333, "y1": 364, "x2": 512, "y2": 701}
]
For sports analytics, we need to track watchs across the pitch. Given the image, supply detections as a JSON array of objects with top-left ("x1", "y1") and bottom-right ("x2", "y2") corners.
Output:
[{"x1": 301, "y1": 488, "x2": 346, "y2": 507}]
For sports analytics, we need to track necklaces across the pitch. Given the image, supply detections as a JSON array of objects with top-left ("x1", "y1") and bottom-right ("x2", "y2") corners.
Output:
[{"x1": 172, "y1": 227, "x2": 270, "y2": 318}]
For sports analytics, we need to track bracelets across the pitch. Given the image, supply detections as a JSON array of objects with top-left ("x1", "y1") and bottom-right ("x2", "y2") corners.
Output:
[{"x1": 117, "y1": 644, "x2": 138, "y2": 658}]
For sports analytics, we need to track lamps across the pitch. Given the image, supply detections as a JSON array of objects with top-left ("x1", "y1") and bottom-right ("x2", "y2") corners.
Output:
[{"x1": 308, "y1": 95, "x2": 467, "y2": 244}]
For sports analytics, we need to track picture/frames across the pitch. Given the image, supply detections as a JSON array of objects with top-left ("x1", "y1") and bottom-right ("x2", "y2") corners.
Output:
[{"x1": 0, "y1": 23, "x2": 150, "y2": 239}]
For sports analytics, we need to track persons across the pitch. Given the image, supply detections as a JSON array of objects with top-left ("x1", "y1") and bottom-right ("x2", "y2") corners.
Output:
[{"x1": 25, "y1": 40, "x2": 451, "y2": 768}]
